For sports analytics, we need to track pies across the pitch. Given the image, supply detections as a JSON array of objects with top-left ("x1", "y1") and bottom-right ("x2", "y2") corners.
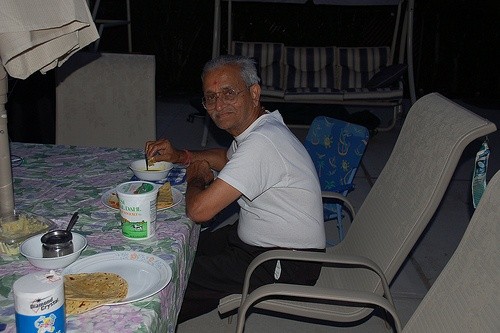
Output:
[
  {"x1": 63, "y1": 272, "x2": 128, "y2": 301},
  {"x1": 157, "y1": 182, "x2": 174, "y2": 208},
  {"x1": 64, "y1": 299, "x2": 114, "y2": 316}
]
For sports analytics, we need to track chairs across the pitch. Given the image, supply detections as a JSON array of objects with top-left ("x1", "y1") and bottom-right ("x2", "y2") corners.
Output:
[
  {"x1": 303, "y1": 116, "x2": 370, "y2": 247},
  {"x1": 403, "y1": 169, "x2": 500, "y2": 333},
  {"x1": 55, "y1": 51, "x2": 156, "y2": 148},
  {"x1": 219, "y1": 91, "x2": 498, "y2": 333}
]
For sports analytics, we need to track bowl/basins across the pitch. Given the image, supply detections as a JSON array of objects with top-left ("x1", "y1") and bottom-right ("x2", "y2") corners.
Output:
[
  {"x1": 128, "y1": 158, "x2": 173, "y2": 181},
  {"x1": 18, "y1": 231, "x2": 88, "y2": 270}
]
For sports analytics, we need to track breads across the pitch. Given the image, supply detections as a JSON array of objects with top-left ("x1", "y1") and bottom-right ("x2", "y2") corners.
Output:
[{"x1": 148, "y1": 157, "x2": 156, "y2": 167}]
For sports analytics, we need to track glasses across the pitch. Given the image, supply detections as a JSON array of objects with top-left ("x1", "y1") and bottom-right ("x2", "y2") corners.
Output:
[{"x1": 202, "y1": 84, "x2": 254, "y2": 110}]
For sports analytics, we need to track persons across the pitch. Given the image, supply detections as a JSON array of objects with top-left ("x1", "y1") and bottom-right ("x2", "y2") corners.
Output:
[{"x1": 145, "y1": 57, "x2": 327, "y2": 324}]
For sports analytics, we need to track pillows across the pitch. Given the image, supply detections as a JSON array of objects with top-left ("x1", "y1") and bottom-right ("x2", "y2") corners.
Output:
[{"x1": 367, "y1": 64, "x2": 408, "y2": 90}]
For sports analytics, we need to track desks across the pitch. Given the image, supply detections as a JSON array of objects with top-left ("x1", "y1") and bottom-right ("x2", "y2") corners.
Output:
[{"x1": 0, "y1": 143, "x2": 218, "y2": 332}]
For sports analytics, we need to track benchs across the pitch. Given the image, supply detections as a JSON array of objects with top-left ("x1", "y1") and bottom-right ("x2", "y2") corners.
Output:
[{"x1": 201, "y1": 0, "x2": 416, "y2": 148}]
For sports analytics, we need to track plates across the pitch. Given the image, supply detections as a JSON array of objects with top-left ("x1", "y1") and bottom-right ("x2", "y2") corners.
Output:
[
  {"x1": 61, "y1": 250, "x2": 173, "y2": 306},
  {"x1": 101, "y1": 183, "x2": 183, "y2": 212}
]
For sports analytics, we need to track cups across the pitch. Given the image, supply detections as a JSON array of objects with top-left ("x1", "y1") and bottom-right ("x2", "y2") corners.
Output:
[{"x1": 116, "y1": 181, "x2": 158, "y2": 240}]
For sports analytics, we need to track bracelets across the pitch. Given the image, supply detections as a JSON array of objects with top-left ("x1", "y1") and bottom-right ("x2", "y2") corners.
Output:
[{"x1": 183, "y1": 148, "x2": 193, "y2": 167}]
